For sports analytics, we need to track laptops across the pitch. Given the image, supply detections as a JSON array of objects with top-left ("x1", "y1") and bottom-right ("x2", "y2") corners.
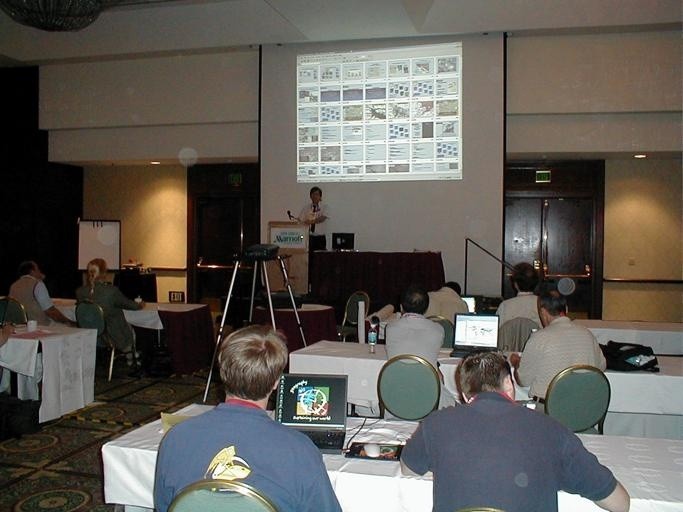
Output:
[
  {"x1": 461, "y1": 297, "x2": 481, "y2": 315},
  {"x1": 275, "y1": 373, "x2": 348, "y2": 454},
  {"x1": 332, "y1": 232, "x2": 354, "y2": 251},
  {"x1": 449, "y1": 313, "x2": 500, "y2": 358}
]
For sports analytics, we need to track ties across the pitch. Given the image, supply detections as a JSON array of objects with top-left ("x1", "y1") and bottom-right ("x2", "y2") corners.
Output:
[{"x1": 311, "y1": 205, "x2": 316, "y2": 232}]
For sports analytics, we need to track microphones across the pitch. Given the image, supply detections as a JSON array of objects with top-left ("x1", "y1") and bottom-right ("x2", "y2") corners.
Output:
[{"x1": 287, "y1": 210, "x2": 292, "y2": 220}]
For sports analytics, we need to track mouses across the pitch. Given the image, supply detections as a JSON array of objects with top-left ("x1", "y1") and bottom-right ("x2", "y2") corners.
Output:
[{"x1": 362, "y1": 442, "x2": 382, "y2": 457}]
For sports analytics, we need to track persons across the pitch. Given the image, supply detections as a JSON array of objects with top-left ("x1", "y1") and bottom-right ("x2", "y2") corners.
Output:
[
  {"x1": 423, "y1": 281, "x2": 469, "y2": 325},
  {"x1": 153, "y1": 325, "x2": 343, "y2": 512},
  {"x1": 399, "y1": 350, "x2": 631, "y2": 512},
  {"x1": 509, "y1": 289, "x2": 608, "y2": 414},
  {"x1": 75, "y1": 258, "x2": 143, "y2": 370},
  {"x1": 8, "y1": 260, "x2": 74, "y2": 326},
  {"x1": 298, "y1": 186, "x2": 332, "y2": 250},
  {"x1": 385, "y1": 287, "x2": 456, "y2": 410},
  {"x1": 496, "y1": 263, "x2": 544, "y2": 329}
]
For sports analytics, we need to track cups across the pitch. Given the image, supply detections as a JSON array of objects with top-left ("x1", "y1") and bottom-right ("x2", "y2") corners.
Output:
[
  {"x1": 27, "y1": 320, "x2": 37, "y2": 333},
  {"x1": 133, "y1": 297, "x2": 142, "y2": 303}
]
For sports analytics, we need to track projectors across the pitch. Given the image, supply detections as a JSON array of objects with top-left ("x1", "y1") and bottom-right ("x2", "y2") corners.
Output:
[{"x1": 240, "y1": 244, "x2": 280, "y2": 259}]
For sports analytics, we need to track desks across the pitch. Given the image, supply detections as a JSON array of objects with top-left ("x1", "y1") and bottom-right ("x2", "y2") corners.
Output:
[
  {"x1": 1, "y1": 327, "x2": 98, "y2": 424},
  {"x1": 100, "y1": 404, "x2": 682, "y2": 511},
  {"x1": 1, "y1": 292, "x2": 211, "y2": 380},
  {"x1": 251, "y1": 288, "x2": 682, "y2": 440}
]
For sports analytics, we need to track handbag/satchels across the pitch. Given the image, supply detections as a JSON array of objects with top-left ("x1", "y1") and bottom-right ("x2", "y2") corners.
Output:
[
  {"x1": 607, "y1": 342, "x2": 657, "y2": 370},
  {"x1": 144, "y1": 344, "x2": 170, "y2": 378}
]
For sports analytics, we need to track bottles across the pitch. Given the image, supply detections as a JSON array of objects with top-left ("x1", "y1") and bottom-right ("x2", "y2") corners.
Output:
[
  {"x1": 368, "y1": 324, "x2": 377, "y2": 355},
  {"x1": 530, "y1": 328, "x2": 537, "y2": 336}
]
